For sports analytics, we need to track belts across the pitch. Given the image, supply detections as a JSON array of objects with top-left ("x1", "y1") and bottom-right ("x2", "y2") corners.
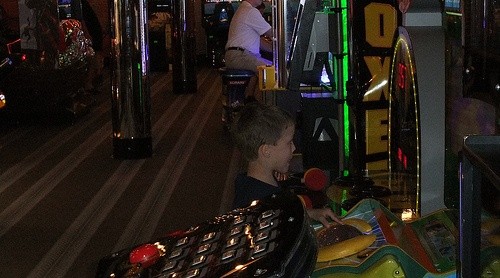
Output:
[{"x1": 227, "y1": 46, "x2": 245, "y2": 52}]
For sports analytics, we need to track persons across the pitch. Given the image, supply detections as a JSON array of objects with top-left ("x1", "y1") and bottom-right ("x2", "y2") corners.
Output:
[
  {"x1": 229, "y1": 103, "x2": 343, "y2": 229},
  {"x1": 226, "y1": 0, "x2": 278, "y2": 105},
  {"x1": 254, "y1": 2, "x2": 266, "y2": 15}
]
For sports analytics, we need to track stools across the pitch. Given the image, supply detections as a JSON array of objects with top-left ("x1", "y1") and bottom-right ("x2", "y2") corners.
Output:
[{"x1": 218, "y1": 67, "x2": 255, "y2": 125}]
